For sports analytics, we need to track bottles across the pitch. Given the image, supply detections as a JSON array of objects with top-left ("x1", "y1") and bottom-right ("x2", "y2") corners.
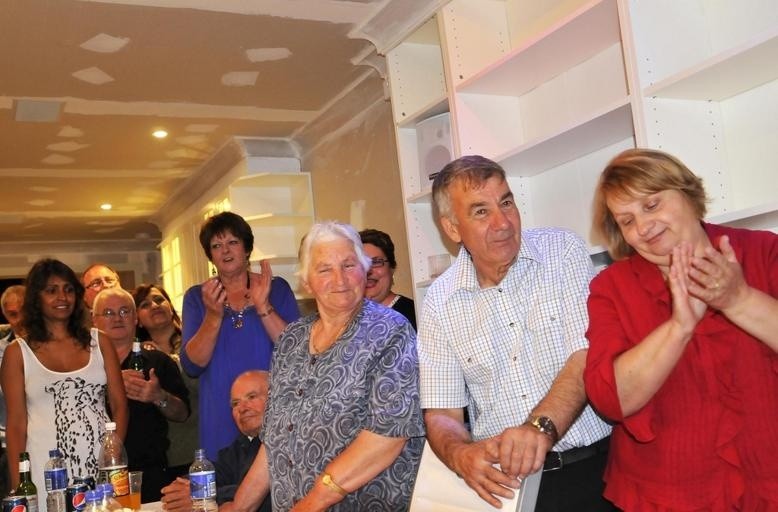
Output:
[
  {"x1": 84, "y1": 483, "x2": 123, "y2": 512},
  {"x1": 95, "y1": 421, "x2": 131, "y2": 512},
  {"x1": 16, "y1": 451, "x2": 39, "y2": 512},
  {"x1": 128, "y1": 336, "x2": 144, "y2": 373},
  {"x1": 43, "y1": 448, "x2": 68, "y2": 512},
  {"x1": 187, "y1": 448, "x2": 219, "y2": 511}
]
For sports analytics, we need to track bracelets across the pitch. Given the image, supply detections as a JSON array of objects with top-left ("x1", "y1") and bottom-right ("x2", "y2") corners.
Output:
[{"x1": 257, "y1": 303, "x2": 275, "y2": 317}]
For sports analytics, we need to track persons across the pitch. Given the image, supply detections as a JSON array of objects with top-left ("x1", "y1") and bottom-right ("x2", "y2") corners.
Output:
[
  {"x1": 180, "y1": 212, "x2": 301, "y2": 462},
  {"x1": 2, "y1": 258, "x2": 200, "y2": 512},
  {"x1": 584, "y1": 149, "x2": 778, "y2": 512},
  {"x1": 162, "y1": 367, "x2": 273, "y2": 512},
  {"x1": 417, "y1": 155, "x2": 620, "y2": 512},
  {"x1": 219, "y1": 222, "x2": 426, "y2": 512},
  {"x1": 357, "y1": 229, "x2": 417, "y2": 333}
]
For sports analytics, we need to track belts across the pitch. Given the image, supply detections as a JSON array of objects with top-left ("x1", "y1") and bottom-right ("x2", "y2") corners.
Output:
[{"x1": 542, "y1": 437, "x2": 609, "y2": 473}]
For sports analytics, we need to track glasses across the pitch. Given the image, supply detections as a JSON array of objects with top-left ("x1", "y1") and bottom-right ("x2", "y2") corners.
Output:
[
  {"x1": 96, "y1": 308, "x2": 133, "y2": 318},
  {"x1": 371, "y1": 259, "x2": 388, "y2": 268}
]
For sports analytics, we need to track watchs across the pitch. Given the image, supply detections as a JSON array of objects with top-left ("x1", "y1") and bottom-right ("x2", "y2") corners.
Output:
[
  {"x1": 321, "y1": 471, "x2": 349, "y2": 497},
  {"x1": 523, "y1": 416, "x2": 559, "y2": 445}
]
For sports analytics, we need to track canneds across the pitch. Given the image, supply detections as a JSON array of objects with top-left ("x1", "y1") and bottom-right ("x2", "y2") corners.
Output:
[
  {"x1": 73, "y1": 476, "x2": 96, "y2": 490},
  {"x1": 2, "y1": 495, "x2": 28, "y2": 512},
  {"x1": 66, "y1": 483, "x2": 89, "y2": 512}
]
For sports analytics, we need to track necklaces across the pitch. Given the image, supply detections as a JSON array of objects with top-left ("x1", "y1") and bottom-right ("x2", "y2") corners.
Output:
[{"x1": 219, "y1": 270, "x2": 251, "y2": 330}]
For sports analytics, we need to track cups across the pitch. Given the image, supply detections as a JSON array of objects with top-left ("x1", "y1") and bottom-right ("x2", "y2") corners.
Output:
[
  {"x1": 428, "y1": 253, "x2": 453, "y2": 277},
  {"x1": 127, "y1": 469, "x2": 145, "y2": 512}
]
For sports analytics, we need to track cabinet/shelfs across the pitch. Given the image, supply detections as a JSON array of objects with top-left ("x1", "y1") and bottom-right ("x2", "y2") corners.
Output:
[
  {"x1": 228, "y1": 171, "x2": 317, "y2": 303},
  {"x1": 346, "y1": 1, "x2": 778, "y2": 337}
]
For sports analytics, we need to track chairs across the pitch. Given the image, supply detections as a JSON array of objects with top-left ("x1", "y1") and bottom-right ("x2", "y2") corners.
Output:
[{"x1": 407, "y1": 438, "x2": 548, "y2": 512}]
